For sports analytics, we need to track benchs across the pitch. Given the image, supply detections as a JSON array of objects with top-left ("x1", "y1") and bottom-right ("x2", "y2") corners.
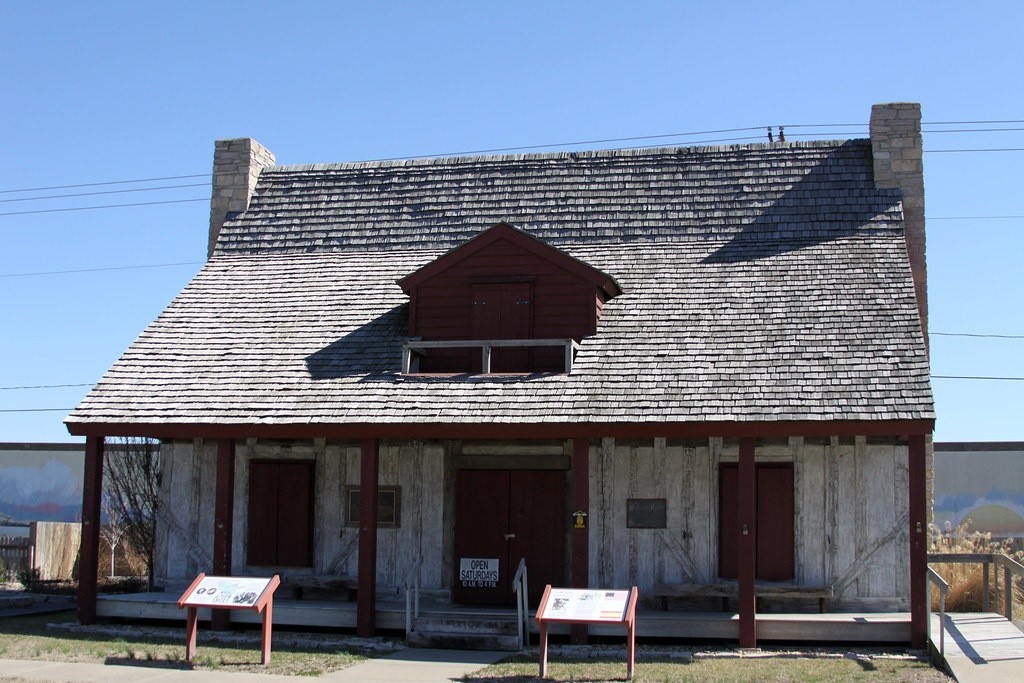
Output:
[
  {"x1": 284, "y1": 575, "x2": 359, "y2": 603},
  {"x1": 652, "y1": 582, "x2": 833, "y2": 614}
]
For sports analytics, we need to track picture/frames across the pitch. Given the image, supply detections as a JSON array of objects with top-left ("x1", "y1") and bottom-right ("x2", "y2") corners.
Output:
[
  {"x1": 343, "y1": 484, "x2": 402, "y2": 529},
  {"x1": 626, "y1": 498, "x2": 667, "y2": 529}
]
